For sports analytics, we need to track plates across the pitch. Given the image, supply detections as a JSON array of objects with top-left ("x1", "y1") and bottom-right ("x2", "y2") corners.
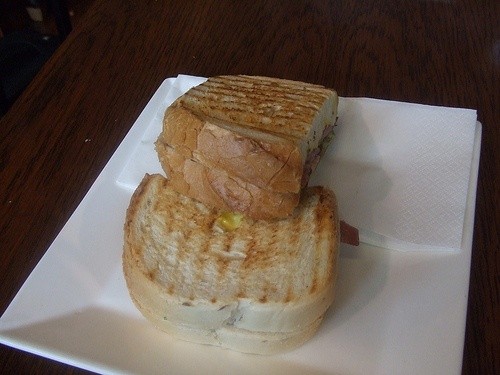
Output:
[{"x1": 0, "y1": 77, "x2": 481, "y2": 375}]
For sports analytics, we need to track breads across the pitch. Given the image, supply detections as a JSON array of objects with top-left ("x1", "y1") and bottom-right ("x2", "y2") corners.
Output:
[
  {"x1": 122, "y1": 171, "x2": 342, "y2": 353},
  {"x1": 155, "y1": 73, "x2": 340, "y2": 217}
]
